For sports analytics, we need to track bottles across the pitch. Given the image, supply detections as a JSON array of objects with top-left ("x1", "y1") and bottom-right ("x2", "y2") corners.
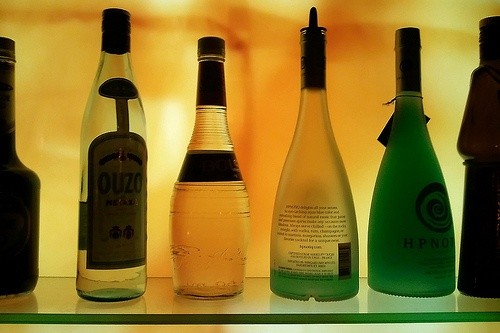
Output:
[
  {"x1": 0, "y1": 36, "x2": 41, "y2": 300},
  {"x1": 457, "y1": 15, "x2": 500, "y2": 299},
  {"x1": 168, "y1": 36, "x2": 252, "y2": 301},
  {"x1": 269, "y1": 6, "x2": 360, "y2": 302},
  {"x1": 75, "y1": 7, "x2": 148, "y2": 304}
]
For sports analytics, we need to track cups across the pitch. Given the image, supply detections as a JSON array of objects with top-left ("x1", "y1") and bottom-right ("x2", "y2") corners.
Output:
[{"x1": 368, "y1": 27, "x2": 456, "y2": 299}]
